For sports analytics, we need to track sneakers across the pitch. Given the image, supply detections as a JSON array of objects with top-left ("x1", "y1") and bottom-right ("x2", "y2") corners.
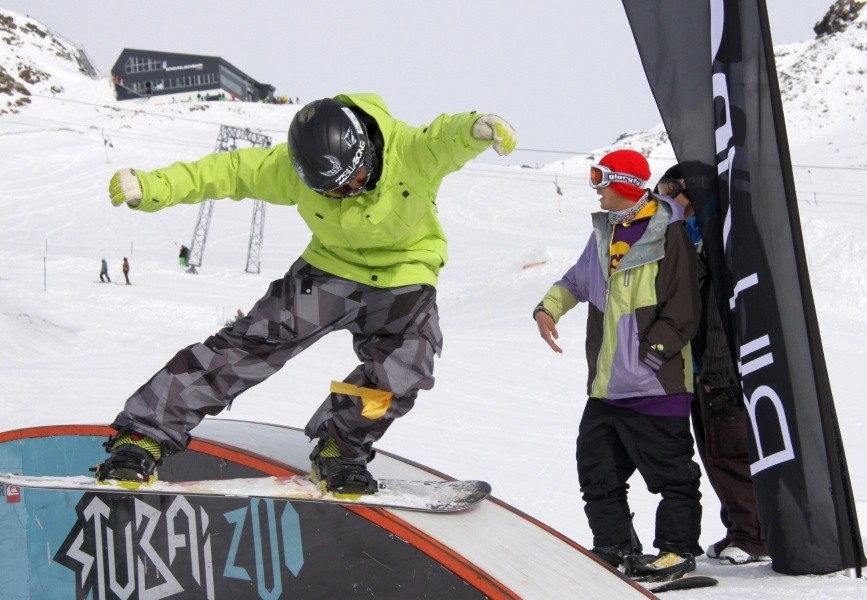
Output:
[
  {"x1": 94, "y1": 449, "x2": 160, "y2": 489},
  {"x1": 309, "y1": 456, "x2": 378, "y2": 495}
]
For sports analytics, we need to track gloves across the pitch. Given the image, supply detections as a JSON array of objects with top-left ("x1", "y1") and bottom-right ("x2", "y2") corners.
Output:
[
  {"x1": 109, "y1": 169, "x2": 143, "y2": 206},
  {"x1": 473, "y1": 113, "x2": 517, "y2": 157}
]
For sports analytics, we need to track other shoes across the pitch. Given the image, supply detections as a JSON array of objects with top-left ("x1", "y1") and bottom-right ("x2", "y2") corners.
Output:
[
  {"x1": 629, "y1": 552, "x2": 693, "y2": 580},
  {"x1": 590, "y1": 543, "x2": 636, "y2": 567},
  {"x1": 707, "y1": 540, "x2": 771, "y2": 564}
]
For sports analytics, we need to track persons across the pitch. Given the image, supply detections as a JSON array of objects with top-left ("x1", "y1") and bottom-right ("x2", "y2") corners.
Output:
[
  {"x1": 179, "y1": 245, "x2": 190, "y2": 266},
  {"x1": 90, "y1": 94, "x2": 519, "y2": 498},
  {"x1": 99, "y1": 259, "x2": 111, "y2": 283},
  {"x1": 651, "y1": 160, "x2": 771, "y2": 568},
  {"x1": 533, "y1": 151, "x2": 705, "y2": 579},
  {"x1": 123, "y1": 257, "x2": 130, "y2": 284}
]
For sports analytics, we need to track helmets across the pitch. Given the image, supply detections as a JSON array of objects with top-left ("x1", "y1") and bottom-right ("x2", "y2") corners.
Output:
[{"x1": 287, "y1": 98, "x2": 367, "y2": 193}]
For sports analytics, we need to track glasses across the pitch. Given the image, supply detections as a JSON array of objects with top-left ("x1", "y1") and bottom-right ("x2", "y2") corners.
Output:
[
  {"x1": 657, "y1": 180, "x2": 684, "y2": 199},
  {"x1": 589, "y1": 164, "x2": 611, "y2": 189},
  {"x1": 326, "y1": 164, "x2": 371, "y2": 199}
]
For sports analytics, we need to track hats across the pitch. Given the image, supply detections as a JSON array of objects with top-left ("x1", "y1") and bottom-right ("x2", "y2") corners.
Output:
[
  {"x1": 599, "y1": 149, "x2": 652, "y2": 202},
  {"x1": 653, "y1": 161, "x2": 722, "y2": 215}
]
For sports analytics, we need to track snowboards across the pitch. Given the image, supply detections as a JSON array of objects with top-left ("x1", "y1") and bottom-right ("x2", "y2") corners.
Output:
[
  {"x1": 634, "y1": 575, "x2": 720, "y2": 595},
  {"x1": 0, "y1": 472, "x2": 493, "y2": 516}
]
